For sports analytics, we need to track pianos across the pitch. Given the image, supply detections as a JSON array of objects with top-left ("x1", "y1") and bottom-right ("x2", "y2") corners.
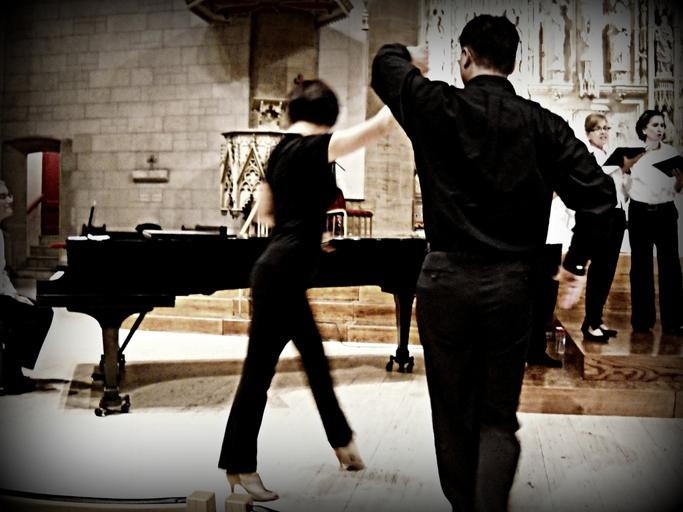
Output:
[{"x1": 35, "y1": 206, "x2": 426, "y2": 416}]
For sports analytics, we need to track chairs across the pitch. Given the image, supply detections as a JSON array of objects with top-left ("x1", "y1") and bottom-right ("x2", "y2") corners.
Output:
[{"x1": 327, "y1": 185, "x2": 373, "y2": 238}]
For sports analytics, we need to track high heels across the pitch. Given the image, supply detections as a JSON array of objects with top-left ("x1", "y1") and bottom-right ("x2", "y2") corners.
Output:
[
  {"x1": 226, "y1": 472, "x2": 278, "y2": 501},
  {"x1": 334, "y1": 446, "x2": 366, "y2": 471}
]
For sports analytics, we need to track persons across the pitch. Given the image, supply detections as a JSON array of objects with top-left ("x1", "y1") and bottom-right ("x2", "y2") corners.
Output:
[
  {"x1": 369, "y1": 14, "x2": 617, "y2": 511},
  {"x1": 629, "y1": 110, "x2": 683, "y2": 335},
  {"x1": 218, "y1": 56, "x2": 426, "y2": 500},
  {"x1": 0, "y1": 181, "x2": 53, "y2": 393},
  {"x1": 582, "y1": 115, "x2": 643, "y2": 344},
  {"x1": 528, "y1": 194, "x2": 563, "y2": 368}
]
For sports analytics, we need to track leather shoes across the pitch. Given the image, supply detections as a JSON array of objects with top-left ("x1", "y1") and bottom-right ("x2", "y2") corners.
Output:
[
  {"x1": 0, "y1": 353, "x2": 31, "y2": 393},
  {"x1": 581, "y1": 320, "x2": 618, "y2": 342}
]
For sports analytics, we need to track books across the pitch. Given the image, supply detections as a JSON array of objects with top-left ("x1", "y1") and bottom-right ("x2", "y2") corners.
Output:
[
  {"x1": 604, "y1": 146, "x2": 649, "y2": 166},
  {"x1": 653, "y1": 155, "x2": 683, "y2": 178}
]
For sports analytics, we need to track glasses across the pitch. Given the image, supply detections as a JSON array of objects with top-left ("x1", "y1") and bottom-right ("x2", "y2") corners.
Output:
[{"x1": 593, "y1": 126, "x2": 611, "y2": 132}]
